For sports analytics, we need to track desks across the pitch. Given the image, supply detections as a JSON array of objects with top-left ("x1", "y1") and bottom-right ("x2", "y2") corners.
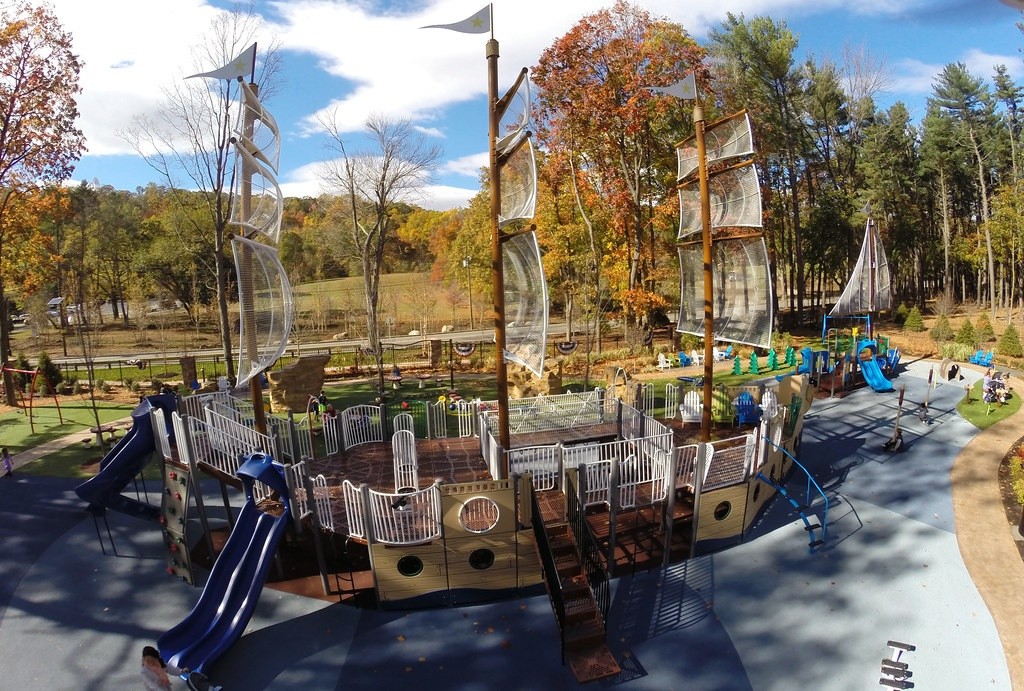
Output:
[
  {"x1": 388, "y1": 376, "x2": 402, "y2": 389},
  {"x1": 416, "y1": 374, "x2": 432, "y2": 388},
  {"x1": 90, "y1": 425, "x2": 113, "y2": 447}
]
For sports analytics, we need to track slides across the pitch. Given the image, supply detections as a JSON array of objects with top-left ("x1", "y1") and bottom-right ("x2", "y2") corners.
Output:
[
  {"x1": 857, "y1": 353, "x2": 892, "y2": 392},
  {"x1": 156, "y1": 499, "x2": 294, "y2": 681},
  {"x1": 74, "y1": 398, "x2": 162, "y2": 522},
  {"x1": 775, "y1": 365, "x2": 809, "y2": 382}
]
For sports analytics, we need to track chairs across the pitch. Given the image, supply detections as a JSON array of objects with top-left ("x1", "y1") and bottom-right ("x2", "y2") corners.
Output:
[
  {"x1": 710, "y1": 390, "x2": 735, "y2": 429},
  {"x1": 678, "y1": 352, "x2": 691, "y2": 367},
  {"x1": 735, "y1": 391, "x2": 761, "y2": 428},
  {"x1": 759, "y1": 391, "x2": 784, "y2": 422},
  {"x1": 713, "y1": 347, "x2": 725, "y2": 362},
  {"x1": 969, "y1": 350, "x2": 983, "y2": 365},
  {"x1": 979, "y1": 351, "x2": 994, "y2": 367},
  {"x1": 691, "y1": 350, "x2": 703, "y2": 366},
  {"x1": 656, "y1": 353, "x2": 671, "y2": 370},
  {"x1": 679, "y1": 390, "x2": 704, "y2": 429},
  {"x1": 667, "y1": 353, "x2": 681, "y2": 368},
  {"x1": 217, "y1": 377, "x2": 230, "y2": 393},
  {"x1": 720, "y1": 345, "x2": 732, "y2": 359}
]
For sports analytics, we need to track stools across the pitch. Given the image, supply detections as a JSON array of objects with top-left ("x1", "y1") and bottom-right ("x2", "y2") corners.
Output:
[
  {"x1": 436, "y1": 378, "x2": 443, "y2": 387},
  {"x1": 107, "y1": 429, "x2": 117, "y2": 438},
  {"x1": 82, "y1": 438, "x2": 92, "y2": 449},
  {"x1": 124, "y1": 426, "x2": 132, "y2": 433},
  {"x1": 449, "y1": 390, "x2": 463, "y2": 403},
  {"x1": 107, "y1": 438, "x2": 117, "y2": 448}
]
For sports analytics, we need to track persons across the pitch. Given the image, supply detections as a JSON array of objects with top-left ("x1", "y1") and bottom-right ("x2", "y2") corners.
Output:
[
  {"x1": 390, "y1": 365, "x2": 403, "y2": 387},
  {"x1": 317, "y1": 391, "x2": 327, "y2": 420},
  {"x1": 0, "y1": 447, "x2": 15, "y2": 478},
  {"x1": 159, "y1": 383, "x2": 179, "y2": 401},
  {"x1": 141, "y1": 645, "x2": 190, "y2": 690},
  {"x1": 322, "y1": 403, "x2": 337, "y2": 441},
  {"x1": 982, "y1": 368, "x2": 1010, "y2": 415},
  {"x1": 312, "y1": 397, "x2": 320, "y2": 422}
]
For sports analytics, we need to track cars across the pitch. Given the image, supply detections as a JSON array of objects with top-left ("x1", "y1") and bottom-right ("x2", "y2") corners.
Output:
[{"x1": 10, "y1": 302, "x2": 93, "y2": 324}]
